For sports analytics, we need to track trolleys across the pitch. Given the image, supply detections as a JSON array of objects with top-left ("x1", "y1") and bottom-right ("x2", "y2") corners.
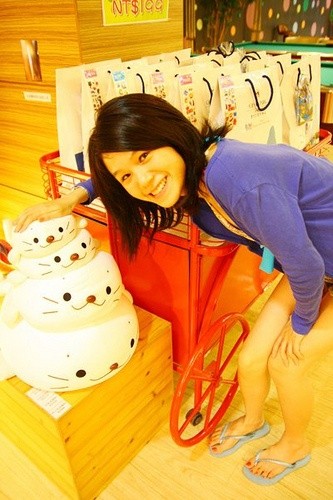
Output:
[{"x1": 37, "y1": 127, "x2": 333, "y2": 448}]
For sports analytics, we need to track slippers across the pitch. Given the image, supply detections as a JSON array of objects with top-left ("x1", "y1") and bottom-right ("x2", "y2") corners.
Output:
[
  {"x1": 210, "y1": 419, "x2": 269, "y2": 458},
  {"x1": 243, "y1": 447, "x2": 310, "y2": 485}
]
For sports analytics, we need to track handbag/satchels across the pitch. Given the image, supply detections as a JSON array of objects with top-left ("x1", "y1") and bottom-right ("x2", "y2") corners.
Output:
[{"x1": 56, "y1": 42, "x2": 322, "y2": 192}]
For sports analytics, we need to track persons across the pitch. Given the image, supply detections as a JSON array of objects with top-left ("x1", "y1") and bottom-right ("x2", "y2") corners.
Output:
[{"x1": 12, "y1": 93, "x2": 333, "y2": 484}]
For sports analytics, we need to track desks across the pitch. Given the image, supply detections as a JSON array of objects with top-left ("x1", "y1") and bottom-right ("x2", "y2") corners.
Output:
[{"x1": 191, "y1": 40, "x2": 333, "y2": 123}]
